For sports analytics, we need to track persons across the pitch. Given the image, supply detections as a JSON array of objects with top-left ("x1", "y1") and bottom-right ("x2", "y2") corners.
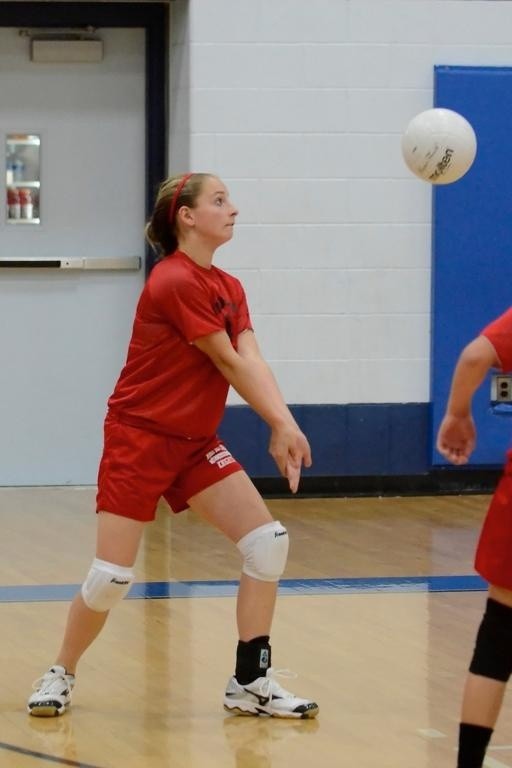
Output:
[
  {"x1": 435, "y1": 306, "x2": 512, "y2": 768},
  {"x1": 25, "y1": 172, "x2": 321, "y2": 720}
]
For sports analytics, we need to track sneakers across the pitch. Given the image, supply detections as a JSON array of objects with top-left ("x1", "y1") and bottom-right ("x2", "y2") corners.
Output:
[
  {"x1": 222, "y1": 668, "x2": 319, "y2": 719},
  {"x1": 26, "y1": 665, "x2": 77, "y2": 718}
]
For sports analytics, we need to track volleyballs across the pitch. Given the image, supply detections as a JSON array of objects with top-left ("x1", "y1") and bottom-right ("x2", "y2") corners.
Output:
[{"x1": 403, "y1": 108, "x2": 477, "y2": 184}]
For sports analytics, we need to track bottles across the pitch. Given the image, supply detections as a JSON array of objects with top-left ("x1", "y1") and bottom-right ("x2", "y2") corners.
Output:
[
  {"x1": 7, "y1": 188, "x2": 34, "y2": 221},
  {"x1": 6, "y1": 149, "x2": 24, "y2": 182}
]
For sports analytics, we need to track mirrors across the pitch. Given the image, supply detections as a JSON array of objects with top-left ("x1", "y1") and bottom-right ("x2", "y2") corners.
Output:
[{"x1": 6, "y1": 133, "x2": 42, "y2": 226}]
